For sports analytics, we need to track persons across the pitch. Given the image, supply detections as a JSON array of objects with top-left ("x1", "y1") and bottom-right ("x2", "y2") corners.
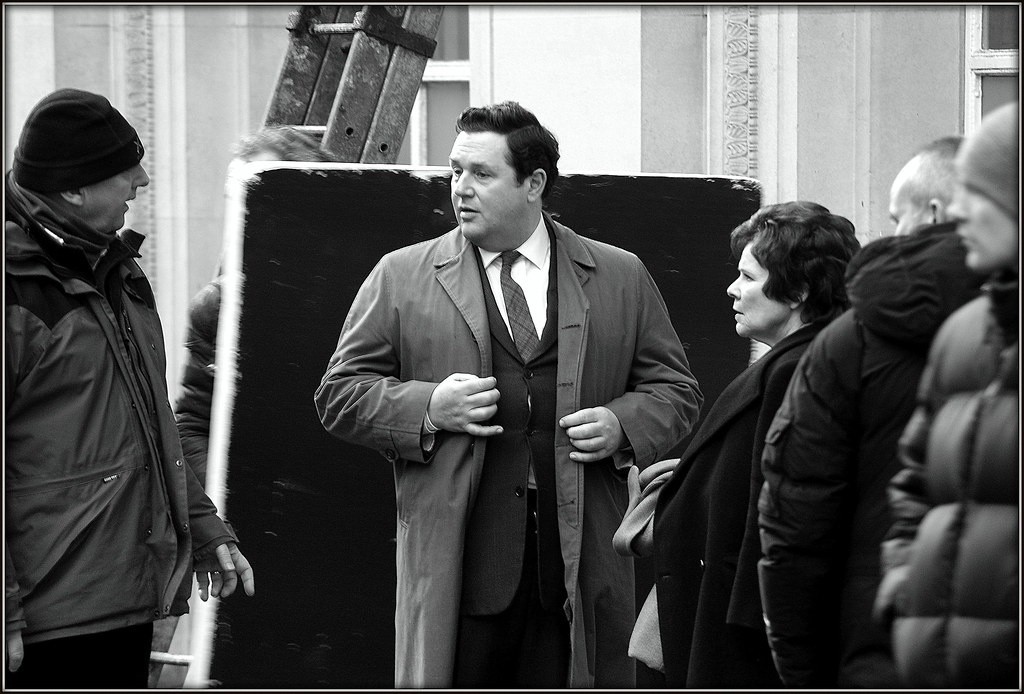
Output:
[
  {"x1": 865, "y1": 102, "x2": 1020, "y2": 688},
  {"x1": 613, "y1": 201, "x2": 863, "y2": 688},
  {"x1": 755, "y1": 137, "x2": 986, "y2": 687},
  {"x1": 171, "y1": 273, "x2": 222, "y2": 490},
  {"x1": 312, "y1": 101, "x2": 704, "y2": 689},
  {"x1": 5, "y1": 88, "x2": 256, "y2": 689}
]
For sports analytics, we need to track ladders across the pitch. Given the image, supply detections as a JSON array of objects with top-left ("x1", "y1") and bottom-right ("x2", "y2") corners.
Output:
[{"x1": 148, "y1": 4, "x2": 450, "y2": 691}]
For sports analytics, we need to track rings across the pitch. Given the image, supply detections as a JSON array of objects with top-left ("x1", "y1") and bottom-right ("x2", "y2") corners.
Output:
[{"x1": 210, "y1": 571, "x2": 225, "y2": 575}]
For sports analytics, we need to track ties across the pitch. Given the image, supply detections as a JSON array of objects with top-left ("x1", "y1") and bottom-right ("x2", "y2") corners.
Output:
[{"x1": 500, "y1": 251, "x2": 539, "y2": 363}]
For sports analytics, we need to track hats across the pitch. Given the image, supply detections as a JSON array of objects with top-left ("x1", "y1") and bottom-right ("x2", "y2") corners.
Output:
[
  {"x1": 955, "y1": 102, "x2": 1018, "y2": 222},
  {"x1": 12, "y1": 87, "x2": 145, "y2": 191}
]
